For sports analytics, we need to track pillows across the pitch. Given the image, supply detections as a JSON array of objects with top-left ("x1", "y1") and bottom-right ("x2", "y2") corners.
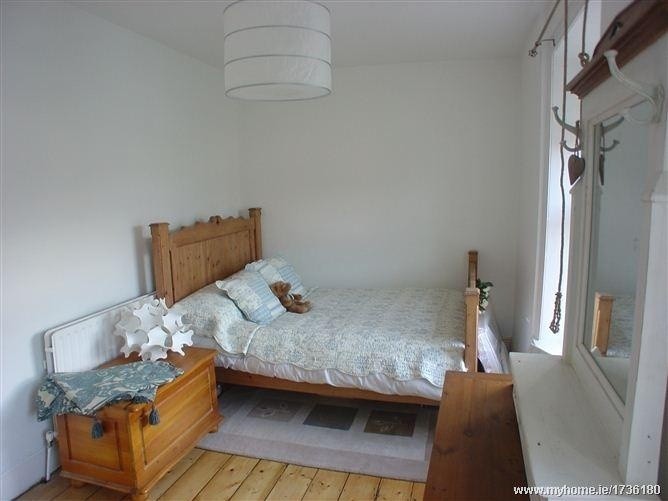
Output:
[
  {"x1": 247, "y1": 255, "x2": 316, "y2": 300},
  {"x1": 212, "y1": 264, "x2": 289, "y2": 325}
]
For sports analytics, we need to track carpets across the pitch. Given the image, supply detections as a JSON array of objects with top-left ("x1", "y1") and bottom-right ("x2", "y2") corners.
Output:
[{"x1": 194, "y1": 383, "x2": 439, "y2": 484}]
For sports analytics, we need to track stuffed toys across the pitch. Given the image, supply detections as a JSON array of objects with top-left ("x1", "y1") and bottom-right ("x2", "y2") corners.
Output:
[{"x1": 269, "y1": 280, "x2": 311, "y2": 313}]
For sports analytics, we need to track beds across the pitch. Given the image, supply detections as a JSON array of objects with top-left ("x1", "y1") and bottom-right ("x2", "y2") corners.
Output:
[
  {"x1": 589, "y1": 289, "x2": 636, "y2": 357},
  {"x1": 146, "y1": 204, "x2": 482, "y2": 421}
]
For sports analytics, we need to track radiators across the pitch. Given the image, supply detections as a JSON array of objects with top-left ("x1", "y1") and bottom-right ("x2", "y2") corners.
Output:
[{"x1": 45, "y1": 291, "x2": 160, "y2": 377}]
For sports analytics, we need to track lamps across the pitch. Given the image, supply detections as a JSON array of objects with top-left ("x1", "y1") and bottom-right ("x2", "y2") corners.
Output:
[{"x1": 221, "y1": 0, "x2": 332, "y2": 102}]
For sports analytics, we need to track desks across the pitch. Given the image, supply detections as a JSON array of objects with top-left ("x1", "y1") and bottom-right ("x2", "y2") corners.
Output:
[{"x1": 422, "y1": 369, "x2": 530, "y2": 501}]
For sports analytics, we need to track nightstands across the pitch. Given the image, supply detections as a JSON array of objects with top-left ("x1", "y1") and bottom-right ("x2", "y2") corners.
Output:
[{"x1": 51, "y1": 346, "x2": 223, "y2": 501}]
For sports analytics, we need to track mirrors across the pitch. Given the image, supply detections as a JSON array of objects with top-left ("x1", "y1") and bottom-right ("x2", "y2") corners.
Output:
[{"x1": 575, "y1": 87, "x2": 664, "y2": 425}]
